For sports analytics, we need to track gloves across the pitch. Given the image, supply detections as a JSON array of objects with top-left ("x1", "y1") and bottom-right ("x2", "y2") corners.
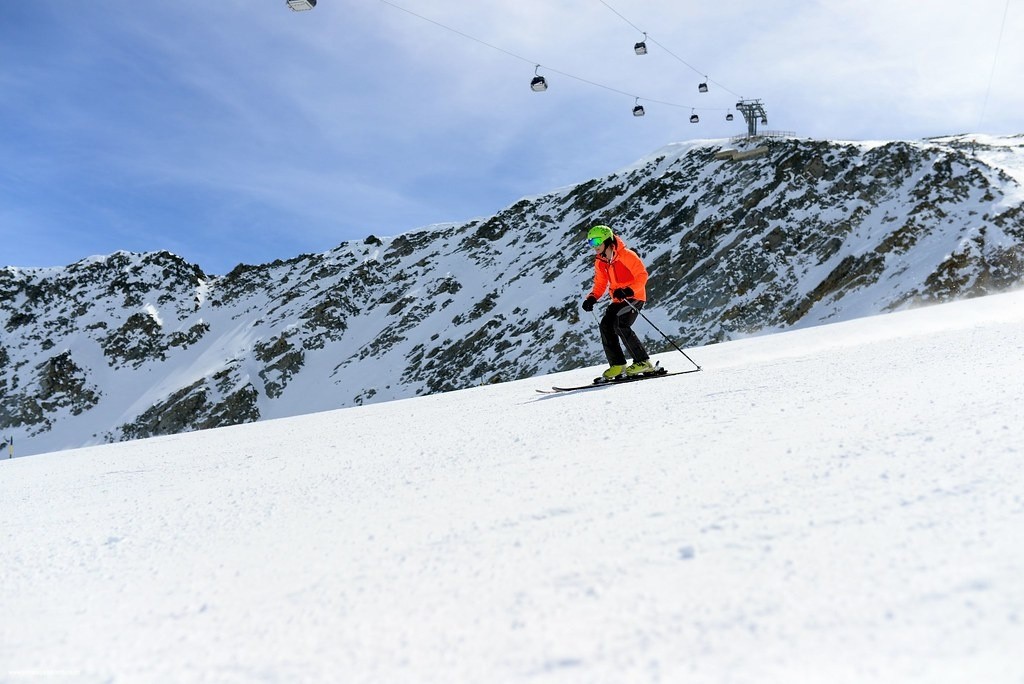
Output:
[
  {"x1": 582, "y1": 295, "x2": 598, "y2": 312},
  {"x1": 612, "y1": 286, "x2": 635, "y2": 303}
]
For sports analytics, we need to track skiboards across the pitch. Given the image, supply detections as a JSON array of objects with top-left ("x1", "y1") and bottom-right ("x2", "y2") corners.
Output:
[{"x1": 535, "y1": 367, "x2": 699, "y2": 395}]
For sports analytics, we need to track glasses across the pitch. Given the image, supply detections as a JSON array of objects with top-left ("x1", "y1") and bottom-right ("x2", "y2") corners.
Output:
[{"x1": 588, "y1": 237, "x2": 610, "y2": 247}]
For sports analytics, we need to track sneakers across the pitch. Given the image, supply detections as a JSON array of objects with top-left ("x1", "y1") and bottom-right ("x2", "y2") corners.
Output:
[
  {"x1": 625, "y1": 361, "x2": 653, "y2": 374},
  {"x1": 602, "y1": 363, "x2": 628, "y2": 377}
]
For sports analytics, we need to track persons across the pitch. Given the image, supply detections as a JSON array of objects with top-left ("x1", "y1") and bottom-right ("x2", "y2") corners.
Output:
[{"x1": 581, "y1": 226, "x2": 653, "y2": 376}]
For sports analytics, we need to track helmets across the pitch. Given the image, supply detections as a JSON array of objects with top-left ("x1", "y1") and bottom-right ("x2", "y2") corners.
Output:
[{"x1": 587, "y1": 225, "x2": 613, "y2": 241}]
